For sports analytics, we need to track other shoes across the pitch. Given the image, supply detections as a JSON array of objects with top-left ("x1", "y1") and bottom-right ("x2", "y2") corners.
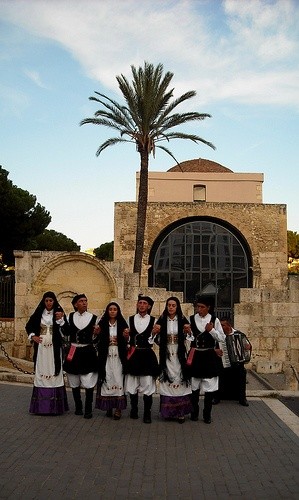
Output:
[
  {"x1": 84, "y1": 406, "x2": 91, "y2": 419},
  {"x1": 131, "y1": 411, "x2": 138, "y2": 420},
  {"x1": 144, "y1": 415, "x2": 150, "y2": 424},
  {"x1": 178, "y1": 415, "x2": 185, "y2": 424},
  {"x1": 213, "y1": 398, "x2": 219, "y2": 404},
  {"x1": 239, "y1": 400, "x2": 248, "y2": 406},
  {"x1": 75, "y1": 408, "x2": 83, "y2": 415},
  {"x1": 192, "y1": 414, "x2": 198, "y2": 421},
  {"x1": 203, "y1": 418, "x2": 210, "y2": 424},
  {"x1": 114, "y1": 410, "x2": 121, "y2": 419},
  {"x1": 106, "y1": 409, "x2": 112, "y2": 417}
]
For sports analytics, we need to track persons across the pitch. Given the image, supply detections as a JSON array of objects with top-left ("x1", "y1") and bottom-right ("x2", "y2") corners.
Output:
[
  {"x1": 185, "y1": 298, "x2": 226, "y2": 424},
  {"x1": 94, "y1": 302, "x2": 129, "y2": 421},
  {"x1": 212, "y1": 316, "x2": 249, "y2": 406},
  {"x1": 149, "y1": 297, "x2": 195, "y2": 424},
  {"x1": 124, "y1": 294, "x2": 159, "y2": 424},
  {"x1": 56, "y1": 294, "x2": 99, "y2": 419},
  {"x1": 25, "y1": 291, "x2": 69, "y2": 416}
]
